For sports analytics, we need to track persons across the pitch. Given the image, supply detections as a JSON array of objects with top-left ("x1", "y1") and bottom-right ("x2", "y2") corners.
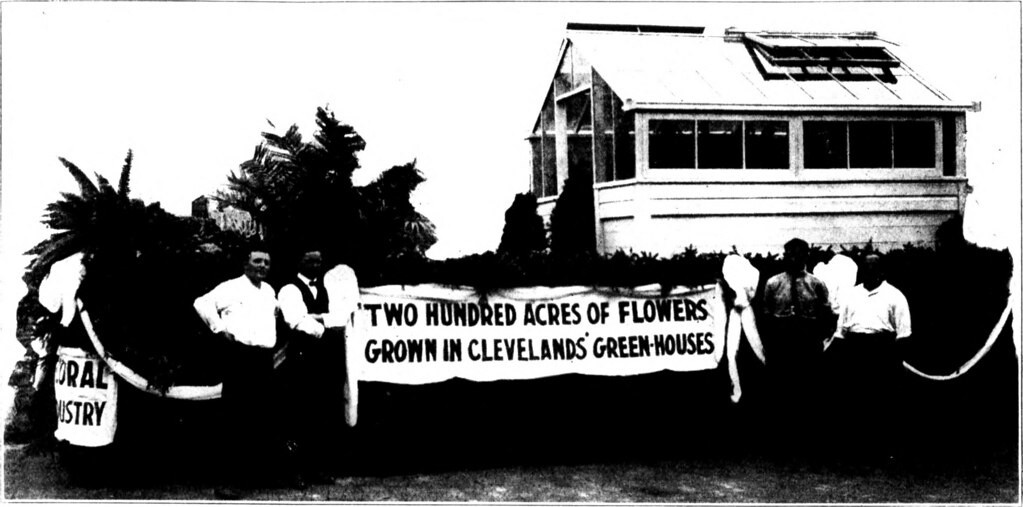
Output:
[
  {"x1": 763, "y1": 240, "x2": 826, "y2": 474},
  {"x1": 276, "y1": 244, "x2": 336, "y2": 490},
  {"x1": 822, "y1": 253, "x2": 913, "y2": 477},
  {"x1": 192, "y1": 245, "x2": 280, "y2": 499}
]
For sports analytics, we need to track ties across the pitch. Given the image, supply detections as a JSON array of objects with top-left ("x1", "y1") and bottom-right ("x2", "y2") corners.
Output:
[{"x1": 790, "y1": 276, "x2": 800, "y2": 316}]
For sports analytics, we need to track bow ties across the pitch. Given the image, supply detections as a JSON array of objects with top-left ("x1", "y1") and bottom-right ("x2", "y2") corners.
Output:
[{"x1": 309, "y1": 280, "x2": 320, "y2": 287}]
